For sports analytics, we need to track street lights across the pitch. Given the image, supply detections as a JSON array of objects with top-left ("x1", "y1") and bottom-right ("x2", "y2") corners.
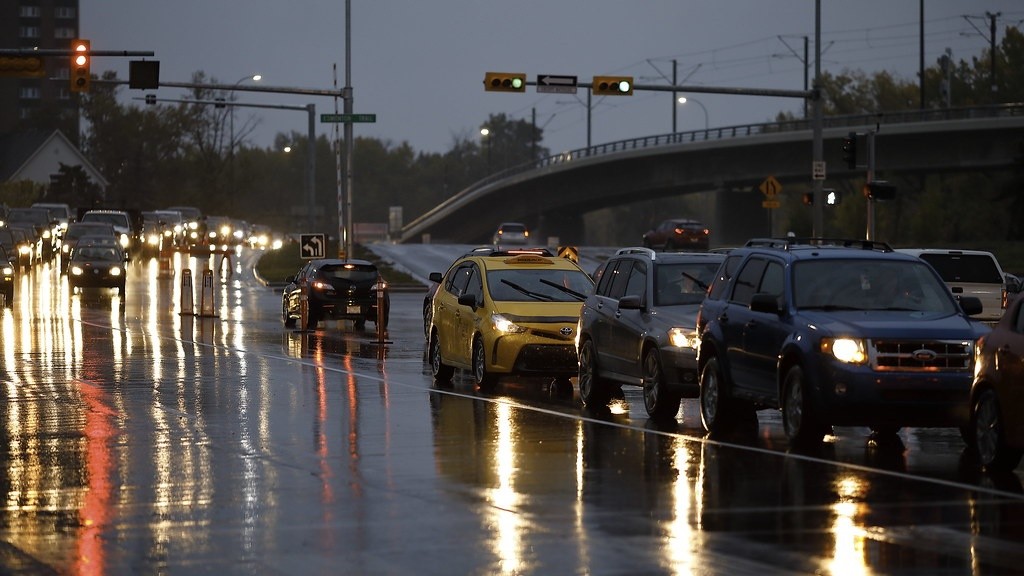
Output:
[
  {"x1": 229, "y1": 73, "x2": 261, "y2": 202},
  {"x1": 481, "y1": 128, "x2": 492, "y2": 173},
  {"x1": 678, "y1": 97, "x2": 709, "y2": 139}
]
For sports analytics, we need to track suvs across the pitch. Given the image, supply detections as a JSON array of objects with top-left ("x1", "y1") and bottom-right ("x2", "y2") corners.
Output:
[
  {"x1": 578, "y1": 243, "x2": 757, "y2": 425},
  {"x1": 692, "y1": 236, "x2": 994, "y2": 453}
]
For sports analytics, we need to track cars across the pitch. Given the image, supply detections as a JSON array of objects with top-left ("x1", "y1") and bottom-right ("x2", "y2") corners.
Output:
[
  {"x1": 281, "y1": 258, "x2": 391, "y2": 331},
  {"x1": 0, "y1": 202, "x2": 286, "y2": 308},
  {"x1": 641, "y1": 217, "x2": 711, "y2": 252},
  {"x1": 492, "y1": 222, "x2": 530, "y2": 247},
  {"x1": 967, "y1": 289, "x2": 1024, "y2": 475},
  {"x1": 426, "y1": 248, "x2": 600, "y2": 396},
  {"x1": 421, "y1": 236, "x2": 1024, "y2": 331}
]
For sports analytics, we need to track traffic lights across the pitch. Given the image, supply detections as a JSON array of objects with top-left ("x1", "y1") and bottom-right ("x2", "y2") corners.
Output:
[
  {"x1": 485, "y1": 72, "x2": 526, "y2": 92},
  {"x1": 864, "y1": 182, "x2": 879, "y2": 199},
  {"x1": 69, "y1": 39, "x2": 90, "y2": 91},
  {"x1": 842, "y1": 138, "x2": 856, "y2": 152},
  {"x1": 823, "y1": 188, "x2": 836, "y2": 207},
  {"x1": 803, "y1": 193, "x2": 813, "y2": 205},
  {"x1": 592, "y1": 76, "x2": 634, "y2": 96}
]
